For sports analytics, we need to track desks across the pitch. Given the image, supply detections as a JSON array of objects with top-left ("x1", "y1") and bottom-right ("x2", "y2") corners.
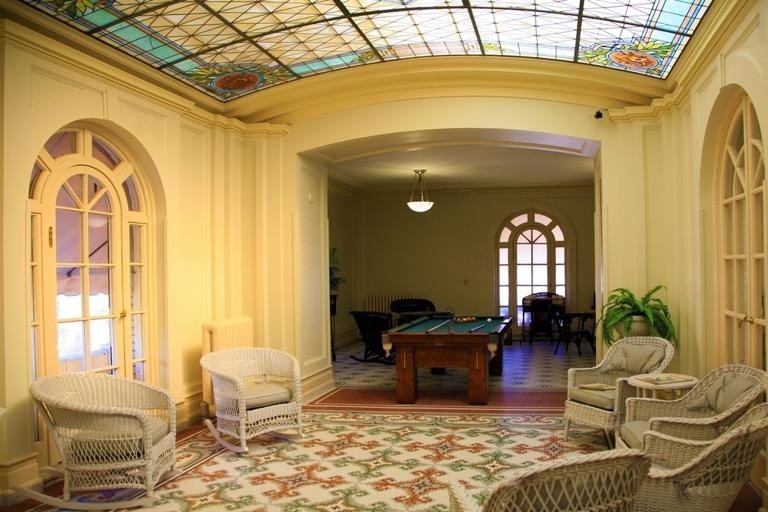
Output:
[
  {"x1": 627, "y1": 370, "x2": 699, "y2": 401},
  {"x1": 385, "y1": 314, "x2": 516, "y2": 407}
]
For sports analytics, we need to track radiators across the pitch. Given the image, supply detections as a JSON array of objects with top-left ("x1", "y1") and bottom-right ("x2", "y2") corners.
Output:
[
  {"x1": 365, "y1": 292, "x2": 415, "y2": 318},
  {"x1": 199, "y1": 314, "x2": 254, "y2": 407}
]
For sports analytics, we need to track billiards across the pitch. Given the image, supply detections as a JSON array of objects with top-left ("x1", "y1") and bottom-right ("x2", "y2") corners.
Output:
[{"x1": 454, "y1": 317, "x2": 476, "y2": 322}]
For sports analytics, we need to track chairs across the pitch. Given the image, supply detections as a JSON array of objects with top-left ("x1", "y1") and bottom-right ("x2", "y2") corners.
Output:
[
  {"x1": 390, "y1": 298, "x2": 438, "y2": 318},
  {"x1": 200, "y1": 347, "x2": 308, "y2": 456},
  {"x1": 560, "y1": 333, "x2": 676, "y2": 452},
  {"x1": 445, "y1": 446, "x2": 654, "y2": 512},
  {"x1": 28, "y1": 370, "x2": 178, "y2": 501},
  {"x1": 630, "y1": 404, "x2": 767, "y2": 512},
  {"x1": 612, "y1": 364, "x2": 768, "y2": 472},
  {"x1": 0, "y1": 483, "x2": 183, "y2": 512},
  {"x1": 520, "y1": 292, "x2": 597, "y2": 360},
  {"x1": 346, "y1": 308, "x2": 396, "y2": 367}
]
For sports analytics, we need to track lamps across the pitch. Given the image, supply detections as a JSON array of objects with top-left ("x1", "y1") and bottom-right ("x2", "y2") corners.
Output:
[{"x1": 405, "y1": 169, "x2": 436, "y2": 214}]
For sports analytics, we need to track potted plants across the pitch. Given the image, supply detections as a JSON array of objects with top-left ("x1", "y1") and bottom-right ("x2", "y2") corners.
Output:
[
  {"x1": 592, "y1": 279, "x2": 682, "y2": 346},
  {"x1": 328, "y1": 246, "x2": 348, "y2": 316}
]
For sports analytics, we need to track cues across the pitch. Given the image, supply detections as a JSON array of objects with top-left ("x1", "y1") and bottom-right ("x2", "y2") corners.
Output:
[
  {"x1": 424, "y1": 320, "x2": 450, "y2": 332},
  {"x1": 363, "y1": 290, "x2": 416, "y2": 327},
  {"x1": 469, "y1": 324, "x2": 487, "y2": 331}
]
